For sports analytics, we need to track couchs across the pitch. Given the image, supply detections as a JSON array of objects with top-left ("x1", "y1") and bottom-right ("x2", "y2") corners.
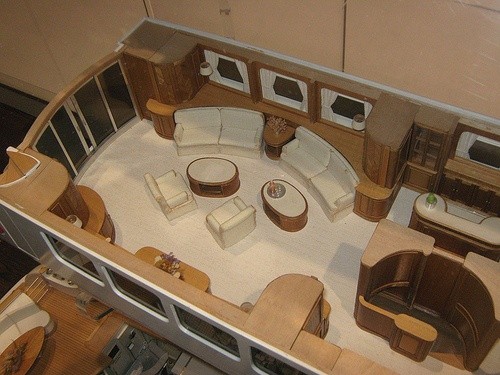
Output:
[
  {"x1": 279, "y1": 126, "x2": 360, "y2": 224},
  {"x1": 172, "y1": 106, "x2": 265, "y2": 160},
  {"x1": 205, "y1": 195, "x2": 257, "y2": 250},
  {"x1": 144, "y1": 169, "x2": 198, "y2": 221}
]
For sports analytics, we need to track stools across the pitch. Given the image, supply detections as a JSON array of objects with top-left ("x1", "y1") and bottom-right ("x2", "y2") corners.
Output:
[{"x1": 389, "y1": 313, "x2": 438, "y2": 362}]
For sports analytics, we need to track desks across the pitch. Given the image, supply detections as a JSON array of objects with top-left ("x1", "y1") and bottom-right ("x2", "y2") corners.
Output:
[
  {"x1": 261, "y1": 178, "x2": 309, "y2": 233},
  {"x1": 134, "y1": 246, "x2": 210, "y2": 293},
  {"x1": 186, "y1": 157, "x2": 241, "y2": 198}
]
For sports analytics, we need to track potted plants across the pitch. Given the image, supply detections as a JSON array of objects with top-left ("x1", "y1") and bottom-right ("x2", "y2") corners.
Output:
[{"x1": 352, "y1": 114, "x2": 366, "y2": 131}]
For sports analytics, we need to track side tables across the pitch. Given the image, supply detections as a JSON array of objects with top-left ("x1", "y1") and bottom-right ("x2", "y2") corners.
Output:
[{"x1": 264, "y1": 121, "x2": 296, "y2": 161}]
